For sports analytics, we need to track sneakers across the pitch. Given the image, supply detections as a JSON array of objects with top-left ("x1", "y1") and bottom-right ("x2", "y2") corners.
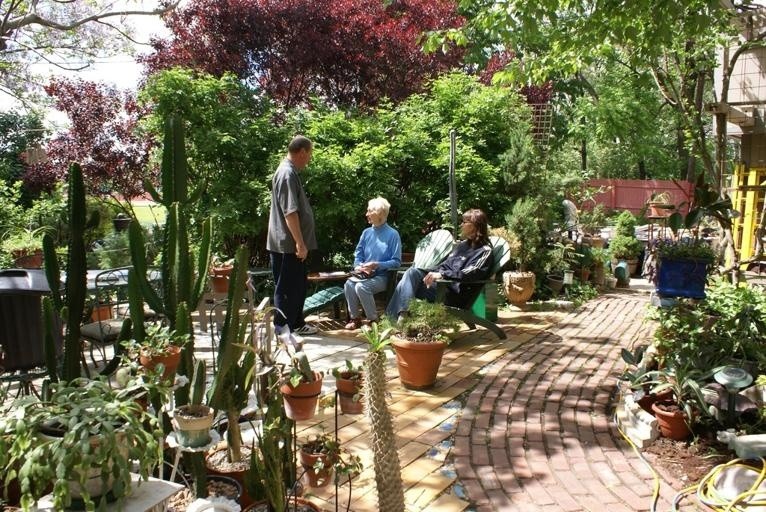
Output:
[
  {"x1": 274, "y1": 332, "x2": 304, "y2": 345},
  {"x1": 397, "y1": 314, "x2": 408, "y2": 325},
  {"x1": 293, "y1": 323, "x2": 318, "y2": 334},
  {"x1": 364, "y1": 319, "x2": 379, "y2": 328},
  {"x1": 346, "y1": 319, "x2": 362, "y2": 329}
]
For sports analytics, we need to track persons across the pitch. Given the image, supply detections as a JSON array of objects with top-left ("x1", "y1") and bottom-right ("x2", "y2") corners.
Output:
[
  {"x1": 344, "y1": 196, "x2": 402, "y2": 330},
  {"x1": 265, "y1": 136, "x2": 320, "y2": 335},
  {"x1": 386, "y1": 207, "x2": 496, "y2": 328}
]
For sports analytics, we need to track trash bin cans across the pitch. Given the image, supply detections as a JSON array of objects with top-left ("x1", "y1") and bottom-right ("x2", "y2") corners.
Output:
[{"x1": 0, "y1": 268, "x2": 51, "y2": 372}]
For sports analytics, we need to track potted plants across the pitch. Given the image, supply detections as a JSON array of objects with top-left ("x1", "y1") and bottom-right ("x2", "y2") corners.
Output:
[
  {"x1": 243, "y1": 374, "x2": 322, "y2": 510},
  {"x1": 278, "y1": 332, "x2": 324, "y2": 418},
  {"x1": 615, "y1": 175, "x2": 765, "y2": 440},
  {"x1": 300, "y1": 434, "x2": 341, "y2": 487},
  {"x1": 502, "y1": 197, "x2": 547, "y2": 305},
  {"x1": 330, "y1": 358, "x2": 367, "y2": 416},
  {"x1": 389, "y1": 298, "x2": 455, "y2": 392},
  {"x1": 0, "y1": 112, "x2": 267, "y2": 512}
]
